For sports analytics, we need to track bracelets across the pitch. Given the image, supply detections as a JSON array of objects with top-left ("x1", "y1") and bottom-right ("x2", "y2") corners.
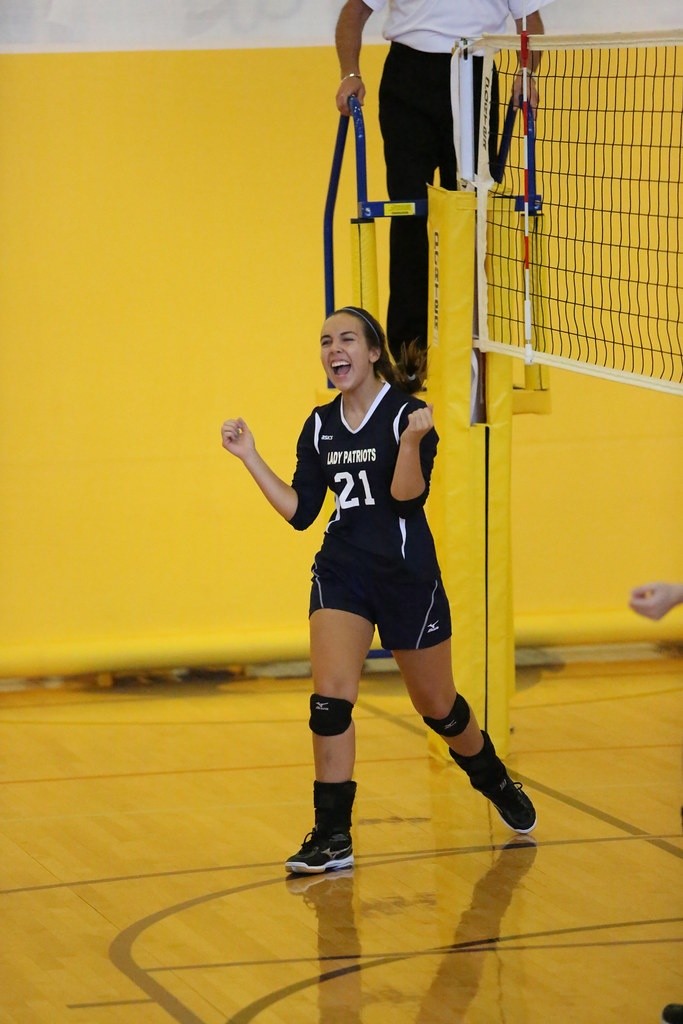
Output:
[
  {"x1": 519, "y1": 68, "x2": 537, "y2": 83},
  {"x1": 341, "y1": 74, "x2": 362, "y2": 82}
]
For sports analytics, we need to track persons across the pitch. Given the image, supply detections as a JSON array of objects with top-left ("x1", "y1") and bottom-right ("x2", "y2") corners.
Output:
[
  {"x1": 335, "y1": 0, "x2": 545, "y2": 396},
  {"x1": 629, "y1": 581, "x2": 683, "y2": 621},
  {"x1": 221, "y1": 306, "x2": 538, "y2": 874}
]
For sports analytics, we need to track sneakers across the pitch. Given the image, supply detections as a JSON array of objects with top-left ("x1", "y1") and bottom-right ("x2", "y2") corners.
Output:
[
  {"x1": 481, "y1": 775, "x2": 537, "y2": 834},
  {"x1": 284, "y1": 827, "x2": 357, "y2": 872}
]
